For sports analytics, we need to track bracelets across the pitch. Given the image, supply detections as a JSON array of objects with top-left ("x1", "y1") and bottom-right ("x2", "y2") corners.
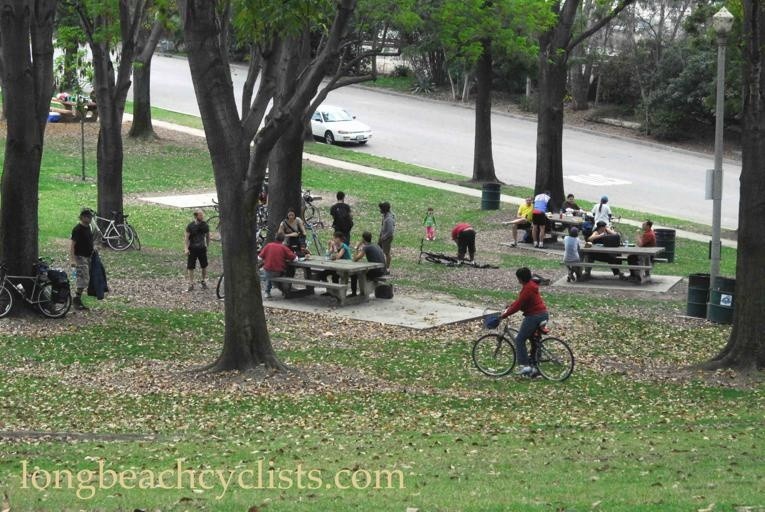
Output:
[{"x1": 354, "y1": 247, "x2": 358, "y2": 250}]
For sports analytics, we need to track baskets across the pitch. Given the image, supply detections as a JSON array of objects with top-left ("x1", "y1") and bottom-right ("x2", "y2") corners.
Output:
[{"x1": 482, "y1": 307, "x2": 502, "y2": 329}]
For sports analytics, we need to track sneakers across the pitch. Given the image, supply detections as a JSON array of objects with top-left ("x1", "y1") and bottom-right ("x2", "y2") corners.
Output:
[
  {"x1": 188, "y1": 283, "x2": 194, "y2": 291},
  {"x1": 201, "y1": 280, "x2": 208, "y2": 289},
  {"x1": 73, "y1": 297, "x2": 89, "y2": 310},
  {"x1": 515, "y1": 366, "x2": 538, "y2": 379}
]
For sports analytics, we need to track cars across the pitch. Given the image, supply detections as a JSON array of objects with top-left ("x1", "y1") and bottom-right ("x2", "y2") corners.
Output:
[{"x1": 310, "y1": 106, "x2": 377, "y2": 148}]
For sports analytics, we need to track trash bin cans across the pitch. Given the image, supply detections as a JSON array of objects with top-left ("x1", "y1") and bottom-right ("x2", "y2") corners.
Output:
[
  {"x1": 687, "y1": 274, "x2": 737, "y2": 325},
  {"x1": 709, "y1": 241, "x2": 722, "y2": 260}
]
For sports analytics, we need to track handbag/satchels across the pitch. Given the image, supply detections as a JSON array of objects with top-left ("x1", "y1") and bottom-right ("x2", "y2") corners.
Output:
[
  {"x1": 48, "y1": 267, "x2": 71, "y2": 304},
  {"x1": 604, "y1": 234, "x2": 620, "y2": 246},
  {"x1": 376, "y1": 283, "x2": 394, "y2": 298}
]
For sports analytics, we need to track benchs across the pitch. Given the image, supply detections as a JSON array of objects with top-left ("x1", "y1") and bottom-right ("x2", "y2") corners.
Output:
[
  {"x1": 544, "y1": 228, "x2": 572, "y2": 239},
  {"x1": 612, "y1": 256, "x2": 669, "y2": 267},
  {"x1": 270, "y1": 276, "x2": 349, "y2": 306},
  {"x1": 580, "y1": 261, "x2": 652, "y2": 284}
]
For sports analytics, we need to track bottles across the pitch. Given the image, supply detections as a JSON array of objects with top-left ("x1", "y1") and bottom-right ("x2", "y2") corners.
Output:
[
  {"x1": 625, "y1": 240, "x2": 629, "y2": 249},
  {"x1": 559, "y1": 209, "x2": 563, "y2": 219},
  {"x1": 324, "y1": 248, "x2": 329, "y2": 259}
]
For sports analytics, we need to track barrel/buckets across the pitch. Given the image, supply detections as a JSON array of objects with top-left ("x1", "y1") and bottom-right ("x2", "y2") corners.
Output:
[
  {"x1": 707, "y1": 275, "x2": 737, "y2": 325},
  {"x1": 687, "y1": 273, "x2": 711, "y2": 319}
]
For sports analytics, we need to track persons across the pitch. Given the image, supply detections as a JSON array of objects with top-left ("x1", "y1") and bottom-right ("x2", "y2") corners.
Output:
[
  {"x1": 561, "y1": 193, "x2": 587, "y2": 228},
  {"x1": 183, "y1": 210, "x2": 210, "y2": 292},
  {"x1": 498, "y1": 267, "x2": 551, "y2": 379},
  {"x1": 377, "y1": 201, "x2": 397, "y2": 275},
  {"x1": 329, "y1": 190, "x2": 353, "y2": 246},
  {"x1": 531, "y1": 190, "x2": 554, "y2": 248},
  {"x1": 587, "y1": 220, "x2": 628, "y2": 281},
  {"x1": 563, "y1": 227, "x2": 583, "y2": 282},
  {"x1": 451, "y1": 222, "x2": 476, "y2": 261},
  {"x1": 258, "y1": 232, "x2": 299, "y2": 298},
  {"x1": 628, "y1": 219, "x2": 656, "y2": 280},
  {"x1": 277, "y1": 207, "x2": 308, "y2": 256},
  {"x1": 346, "y1": 232, "x2": 386, "y2": 297},
  {"x1": 591, "y1": 195, "x2": 613, "y2": 232},
  {"x1": 70, "y1": 209, "x2": 93, "y2": 310},
  {"x1": 422, "y1": 207, "x2": 437, "y2": 241},
  {"x1": 319, "y1": 231, "x2": 352, "y2": 296},
  {"x1": 501, "y1": 196, "x2": 533, "y2": 247}
]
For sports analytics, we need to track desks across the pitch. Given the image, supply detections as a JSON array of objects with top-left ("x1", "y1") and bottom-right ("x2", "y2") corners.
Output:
[
  {"x1": 579, "y1": 244, "x2": 666, "y2": 285},
  {"x1": 540, "y1": 212, "x2": 591, "y2": 231},
  {"x1": 289, "y1": 257, "x2": 385, "y2": 305}
]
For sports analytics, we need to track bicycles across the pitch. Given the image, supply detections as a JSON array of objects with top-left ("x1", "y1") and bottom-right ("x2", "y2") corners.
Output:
[
  {"x1": 216, "y1": 228, "x2": 273, "y2": 300},
  {"x1": 86, "y1": 208, "x2": 134, "y2": 251},
  {"x1": 202, "y1": 191, "x2": 327, "y2": 255},
  {"x1": 120, "y1": 212, "x2": 141, "y2": 251},
  {"x1": 470, "y1": 303, "x2": 576, "y2": 383},
  {"x1": 416, "y1": 237, "x2": 501, "y2": 269},
  {"x1": 0, "y1": 257, "x2": 74, "y2": 320}
]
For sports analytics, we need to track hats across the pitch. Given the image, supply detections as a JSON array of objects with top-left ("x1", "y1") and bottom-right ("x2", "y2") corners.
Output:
[
  {"x1": 592, "y1": 221, "x2": 607, "y2": 232},
  {"x1": 80, "y1": 210, "x2": 94, "y2": 218}
]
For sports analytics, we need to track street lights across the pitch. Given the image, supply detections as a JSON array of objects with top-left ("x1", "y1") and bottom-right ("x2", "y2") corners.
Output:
[{"x1": 703, "y1": 3, "x2": 738, "y2": 325}]
